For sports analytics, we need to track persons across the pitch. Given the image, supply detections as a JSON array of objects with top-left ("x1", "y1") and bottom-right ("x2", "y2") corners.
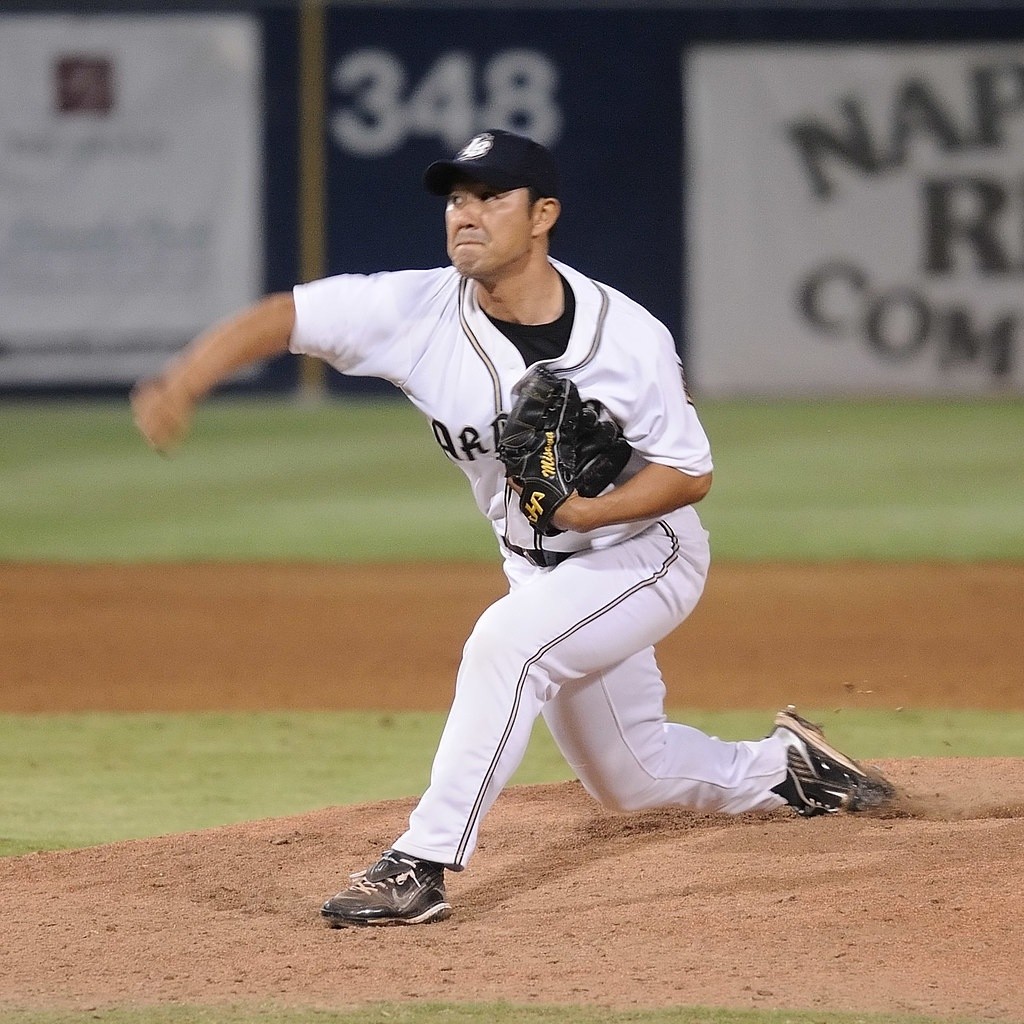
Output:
[{"x1": 129, "y1": 129, "x2": 891, "y2": 922}]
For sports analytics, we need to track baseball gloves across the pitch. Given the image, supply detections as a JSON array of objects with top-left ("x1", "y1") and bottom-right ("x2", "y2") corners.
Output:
[{"x1": 494, "y1": 367, "x2": 637, "y2": 538}]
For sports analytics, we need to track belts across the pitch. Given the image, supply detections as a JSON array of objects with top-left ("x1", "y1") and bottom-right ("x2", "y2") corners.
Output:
[{"x1": 502, "y1": 536, "x2": 576, "y2": 567}]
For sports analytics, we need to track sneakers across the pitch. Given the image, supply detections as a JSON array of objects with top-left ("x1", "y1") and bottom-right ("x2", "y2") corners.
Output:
[
  {"x1": 321, "y1": 849, "x2": 452, "y2": 924},
  {"x1": 763, "y1": 710, "x2": 893, "y2": 817}
]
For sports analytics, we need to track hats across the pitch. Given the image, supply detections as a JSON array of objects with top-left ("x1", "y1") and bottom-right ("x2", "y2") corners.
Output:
[{"x1": 422, "y1": 129, "x2": 557, "y2": 195}]
